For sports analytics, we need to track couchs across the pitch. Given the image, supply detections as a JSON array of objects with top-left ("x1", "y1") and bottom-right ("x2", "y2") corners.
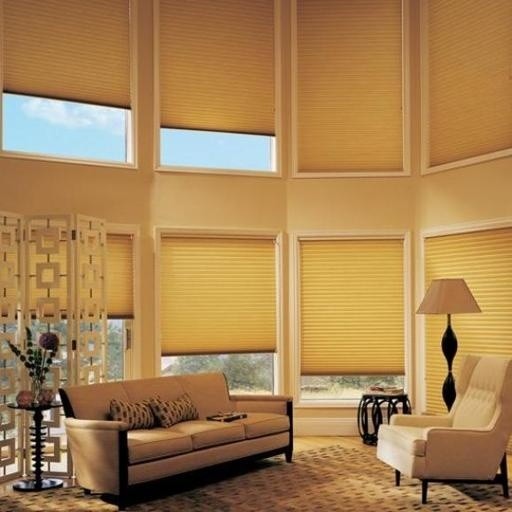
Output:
[{"x1": 58, "y1": 371, "x2": 292, "y2": 511}]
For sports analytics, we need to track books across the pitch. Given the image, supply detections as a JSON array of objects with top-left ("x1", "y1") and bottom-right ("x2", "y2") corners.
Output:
[
  {"x1": 368, "y1": 385, "x2": 404, "y2": 395},
  {"x1": 206, "y1": 411, "x2": 248, "y2": 423}
]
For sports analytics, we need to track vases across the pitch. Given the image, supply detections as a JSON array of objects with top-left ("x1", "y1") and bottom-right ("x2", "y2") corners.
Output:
[{"x1": 17, "y1": 388, "x2": 54, "y2": 404}]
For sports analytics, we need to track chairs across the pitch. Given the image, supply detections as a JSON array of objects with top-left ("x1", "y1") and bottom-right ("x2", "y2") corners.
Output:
[{"x1": 376, "y1": 357, "x2": 512, "y2": 505}]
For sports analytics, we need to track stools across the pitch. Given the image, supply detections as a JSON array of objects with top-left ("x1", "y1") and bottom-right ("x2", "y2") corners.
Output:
[{"x1": 358, "y1": 394, "x2": 413, "y2": 445}]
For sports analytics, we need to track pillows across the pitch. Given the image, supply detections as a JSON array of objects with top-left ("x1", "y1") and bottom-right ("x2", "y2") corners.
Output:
[{"x1": 109, "y1": 392, "x2": 199, "y2": 430}]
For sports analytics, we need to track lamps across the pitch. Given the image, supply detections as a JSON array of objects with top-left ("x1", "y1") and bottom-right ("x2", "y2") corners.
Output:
[{"x1": 415, "y1": 278, "x2": 481, "y2": 412}]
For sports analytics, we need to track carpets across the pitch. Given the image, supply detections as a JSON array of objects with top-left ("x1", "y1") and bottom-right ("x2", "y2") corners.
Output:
[{"x1": 0, "y1": 444, "x2": 512, "y2": 511}]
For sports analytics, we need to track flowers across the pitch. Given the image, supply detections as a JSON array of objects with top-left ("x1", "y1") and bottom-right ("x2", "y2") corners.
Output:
[{"x1": 6, "y1": 326, "x2": 60, "y2": 389}]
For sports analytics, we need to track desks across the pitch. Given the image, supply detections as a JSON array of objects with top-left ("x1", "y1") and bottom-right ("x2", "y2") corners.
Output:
[{"x1": 8, "y1": 401, "x2": 63, "y2": 491}]
